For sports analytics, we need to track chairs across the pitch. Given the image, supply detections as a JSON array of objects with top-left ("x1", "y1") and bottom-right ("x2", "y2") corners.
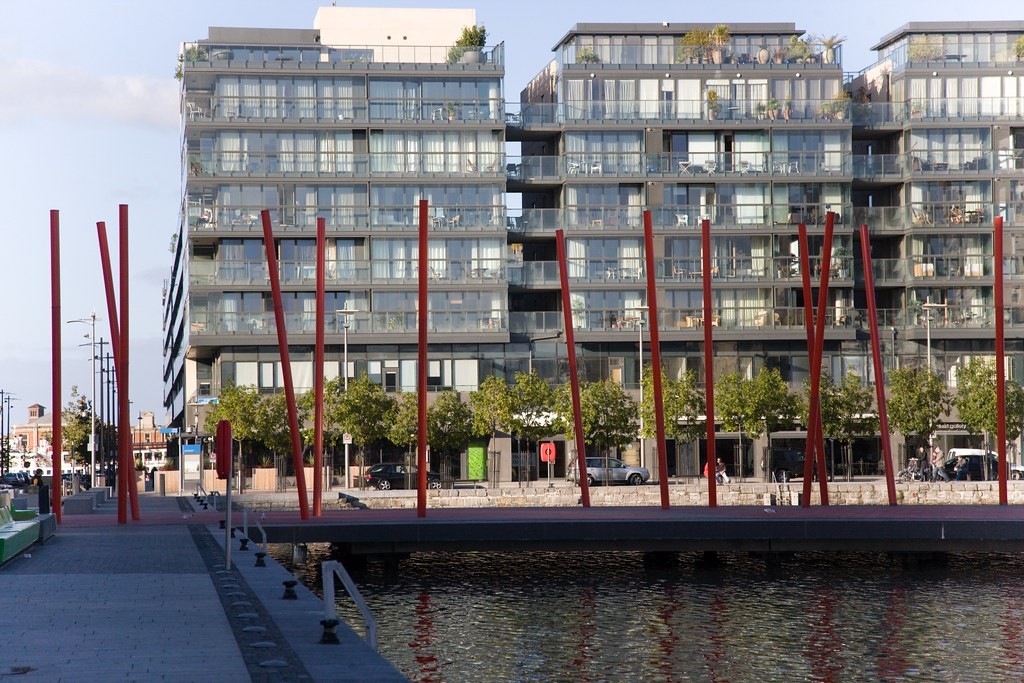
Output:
[
  {"x1": 462, "y1": 266, "x2": 501, "y2": 280},
  {"x1": 912, "y1": 207, "x2": 932, "y2": 224},
  {"x1": 754, "y1": 311, "x2": 781, "y2": 326},
  {"x1": 912, "y1": 156, "x2": 930, "y2": 171},
  {"x1": 772, "y1": 161, "x2": 799, "y2": 174},
  {"x1": 738, "y1": 161, "x2": 752, "y2": 175},
  {"x1": 676, "y1": 214, "x2": 689, "y2": 226},
  {"x1": 711, "y1": 264, "x2": 735, "y2": 279},
  {"x1": 685, "y1": 316, "x2": 721, "y2": 328},
  {"x1": 590, "y1": 159, "x2": 602, "y2": 176},
  {"x1": 506, "y1": 216, "x2": 528, "y2": 229},
  {"x1": 607, "y1": 267, "x2": 643, "y2": 280},
  {"x1": 959, "y1": 157, "x2": 985, "y2": 171},
  {"x1": 189, "y1": 102, "x2": 204, "y2": 118},
  {"x1": 833, "y1": 314, "x2": 846, "y2": 325},
  {"x1": 698, "y1": 213, "x2": 710, "y2": 225},
  {"x1": 507, "y1": 164, "x2": 518, "y2": 176},
  {"x1": 567, "y1": 161, "x2": 579, "y2": 176},
  {"x1": 704, "y1": 159, "x2": 717, "y2": 175},
  {"x1": 446, "y1": 215, "x2": 460, "y2": 228},
  {"x1": 952, "y1": 209, "x2": 985, "y2": 224}
]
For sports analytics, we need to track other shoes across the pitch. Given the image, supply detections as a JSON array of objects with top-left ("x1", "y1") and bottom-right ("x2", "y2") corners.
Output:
[
  {"x1": 931, "y1": 480, "x2": 935, "y2": 483},
  {"x1": 945, "y1": 480, "x2": 950, "y2": 483},
  {"x1": 728, "y1": 479, "x2": 731, "y2": 484}
]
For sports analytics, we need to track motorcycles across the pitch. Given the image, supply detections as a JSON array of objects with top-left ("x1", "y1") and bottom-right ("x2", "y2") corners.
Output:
[{"x1": 896, "y1": 447, "x2": 945, "y2": 484}]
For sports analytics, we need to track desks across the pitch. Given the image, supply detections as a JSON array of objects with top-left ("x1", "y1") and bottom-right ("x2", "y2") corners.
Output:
[
  {"x1": 728, "y1": 107, "x2": 739, "y2": 120},
  {"x1": 934, "y1": 163, "x2": 948, "y2": 172},
  {"x1": 579, "y1": 161, "x2": 589, "y2": 175},
  {"x1": 679, "y1": 161, "x2": 691, "y2": 174},
  {"x1": 788, "y1": 213, "x2": 801, "y2": 223},
  {"x1": 914, "y1": 263, "x2": 934, "y2": 277},
  {"x1": 688, "y1": 271, "x2": 703, "y2": 279},
  {"x1": 471, "y1": 268, "x2": 489, "y2": 281},
  {"x1": 963, "y1": 211, "x2": 980, "y2": 224}
]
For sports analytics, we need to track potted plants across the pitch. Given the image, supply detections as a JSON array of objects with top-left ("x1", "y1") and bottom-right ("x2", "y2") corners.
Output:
[
  {"x1": 731, "y1": 33, "x2": 847, "y2": 65},
  {"x1": 303, "y1": 450, "x2": 329, "y2": 492},
  {"x1": 828, "y1": 248, "x2": 852, "y2": 278},
  {"x1": 816, "y1": 90, "x2": 873, "y2": 120},
  {"x1": 447, "y1": 102, "x2": 456, "y2": 121},
  {"x1": 910, "y1": 103, "x2": 924, "y2": 119},
  {"x1": 154, "y1": 457, "x2": 179, "y2": 493},
  {"x1": 135, "y1": 458, "x2": 145, "y2": 492},
  {"x1": 212, "y1": 49, "x2": 234, "y2": 60},
  {"x1": 188, "y1": 154, "x2": 203, "y2": 174},
  {"x1": 755, "y1": 97, "x2": 795, "y2": 121},
  {"x1": 676, "y1": 25, "x2": 732, "y2": 65},
  {"x1": 576, "y1": 47, "x2": 601, "y2": 64},
  {"x1": 251, "y1": 453, "x2": 279, "y2": 491},
  {"x1": 704, "y1": 91, "x2": 718, "y2": 120},
  {"x1": 445, "y1": 24, "x2": 488, "y2": 64}
]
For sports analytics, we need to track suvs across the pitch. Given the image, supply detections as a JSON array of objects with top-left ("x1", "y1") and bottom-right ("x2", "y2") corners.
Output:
[
  {"x1": 759, "y1": 446, "x2": 832, "y2": 485},
  {"x1": 567, "y1": 455, "x2": 650, "y2": 488}
]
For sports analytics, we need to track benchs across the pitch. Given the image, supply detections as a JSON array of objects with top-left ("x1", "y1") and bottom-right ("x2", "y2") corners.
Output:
[
  {"x1": 427, "y1": 479, "x2": 479, "y2": 490},
  {"x1": 672, "y1": 474, "x2": 723, "y2": 485}
]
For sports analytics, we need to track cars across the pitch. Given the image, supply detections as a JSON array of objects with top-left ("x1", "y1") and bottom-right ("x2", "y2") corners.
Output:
[
  {"x1": 1, "y1": 471, "x2": 91, "y2": 490},
  {"x1": 366, "y1": 461, "x2": 454, "y2": 489},
  {"x1": 917, "y1": 454, "x2": 999, "y2": 482}
]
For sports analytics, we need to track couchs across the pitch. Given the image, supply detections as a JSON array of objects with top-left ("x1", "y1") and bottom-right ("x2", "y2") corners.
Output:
[{"x1": 0, "y1": 505, "x2": 41, "y2": 566}]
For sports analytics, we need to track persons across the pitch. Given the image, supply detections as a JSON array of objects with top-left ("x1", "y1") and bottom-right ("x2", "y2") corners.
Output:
[
  {"x1": 704, "y1": 457, "x2": 730, "y2": 483},
  {"x1": 31, "y1": 469, "x2": 44, "y2": 489},
  {"x1": 918, "y1": 446, "x2": 971, "y2": 483}
]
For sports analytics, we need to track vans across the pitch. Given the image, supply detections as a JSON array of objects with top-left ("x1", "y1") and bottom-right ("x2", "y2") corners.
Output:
[{"x1": 947, "y1": 447, "x2": 1024, "y2": 480}]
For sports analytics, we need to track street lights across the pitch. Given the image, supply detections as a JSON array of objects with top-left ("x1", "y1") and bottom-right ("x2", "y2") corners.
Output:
[
  {"x1": 66, "y1": 315, "x2": 97, "y2": 488},
  {"x1": 525, "y1": 328, "x2": 566, "y2": 486},
  {"x1": 922, "y1": 297, "x2": 949, "y2": 469},
  {"x1": 87, "y1": 356, "x2": 115, "y2": 484},
  {"x1": 96, "y1": 367, "x2": 117, "y2": 486},
  {"x1": 633, "y1": 308, "x2": 651, "y2": 468},
  {"x1": 335, "y1": 308, "x2": 358, "y2": 489},
  {"x1": 79, "y1": 338, "x2": 109, "y2": 482}
]
